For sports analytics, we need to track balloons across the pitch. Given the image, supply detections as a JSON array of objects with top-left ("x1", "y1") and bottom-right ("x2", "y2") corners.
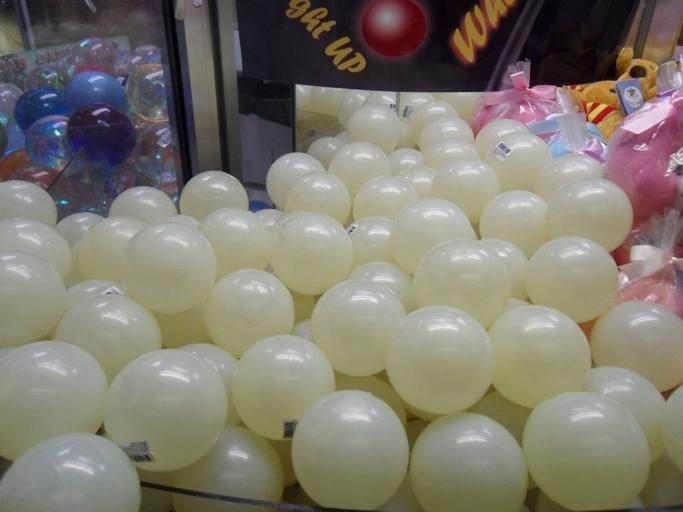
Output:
[
  {"x1": 1, "y1": 39, "x2": 184, "y2": 113},
  {"x1": 0, "y1": 94, "x2": 682, "y2": 511}
]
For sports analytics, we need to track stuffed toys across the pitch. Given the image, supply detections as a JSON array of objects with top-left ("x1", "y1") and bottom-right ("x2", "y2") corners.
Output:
[{"x1": 469, "y1": 47, "x2": 682, "y2": 312}]
